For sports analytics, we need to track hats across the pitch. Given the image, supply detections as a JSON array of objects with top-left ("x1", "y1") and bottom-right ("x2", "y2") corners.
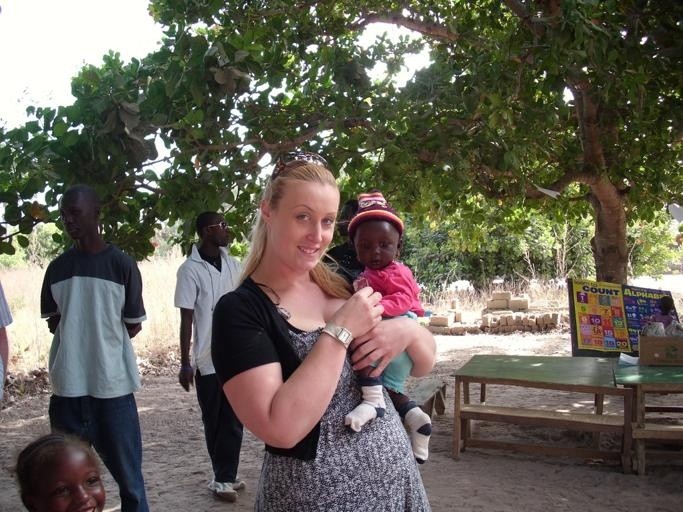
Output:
[{"x1": 347, "y1": 188, "x2": 403, "y2": 239}]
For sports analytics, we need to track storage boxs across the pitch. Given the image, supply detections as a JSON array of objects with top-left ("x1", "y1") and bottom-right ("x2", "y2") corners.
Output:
[{"x1": 637, "y1": 327, "x2": 682, "y2": 367}]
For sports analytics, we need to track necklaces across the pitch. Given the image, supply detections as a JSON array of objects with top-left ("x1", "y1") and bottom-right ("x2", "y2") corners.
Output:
[{"x1": 201, "y1": 243, "x2": 219, "y2": 268}]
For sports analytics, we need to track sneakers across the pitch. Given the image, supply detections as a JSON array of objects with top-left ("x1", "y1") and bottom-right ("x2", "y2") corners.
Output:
[
  {"x1": 232, "y1": 480, "x2": 245, "y2": 490},
  {"x1": 206, "y1": 479, "x2": 236, "y2": 502}
]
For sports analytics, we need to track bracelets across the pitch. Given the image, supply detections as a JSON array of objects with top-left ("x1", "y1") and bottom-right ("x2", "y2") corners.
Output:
[{"x1": 180, "y1": 366, "x2": 193, "y2": 372}]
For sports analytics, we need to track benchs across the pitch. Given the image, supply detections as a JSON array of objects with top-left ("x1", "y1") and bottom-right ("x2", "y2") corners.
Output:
[{"x1": 404, "y1": 379, "x2": 447, "y2": 422}]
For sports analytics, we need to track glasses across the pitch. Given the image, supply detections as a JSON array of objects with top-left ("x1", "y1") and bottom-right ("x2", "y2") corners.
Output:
[
  {"x1": 207, "y1": 221, "x2": 227, "y2": 229},
  {"x1": 270, "y1": 151, "x2": 328, "y2": 179}
]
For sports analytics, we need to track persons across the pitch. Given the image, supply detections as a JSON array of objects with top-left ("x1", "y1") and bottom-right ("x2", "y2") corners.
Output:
[
  {"x1": 173, "y1": 210, "x2": 246, "y2": 502},
  {"x1": 343, "y1": 187, "x2": 433, "y2": 466},
  {"x1": 0, "y1": 278, "x2": 14, "y2": 415},
  {"x1": 39, "y1": 184, "x2": 151, "y2": 512},
  {"x1": 208, "y1": 153, "x2": 438, "y2": 512},
  {"x1": 14, "y1": 433, "x2": 107, "y2": 512},
  {"x1": 646, "y1": 296, "x2": 678, "y2": 329}
]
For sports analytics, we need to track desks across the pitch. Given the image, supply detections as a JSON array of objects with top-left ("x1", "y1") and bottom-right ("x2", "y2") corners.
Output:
[
  {"x1": 449, "y1": 354, "x2": 636, "y2": 475},
  {"x1": 611, "y1": 355, "x2": 681, "y2": 476}
]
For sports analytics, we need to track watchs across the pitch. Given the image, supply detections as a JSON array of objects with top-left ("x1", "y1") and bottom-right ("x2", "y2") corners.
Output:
[{"x1": 320, "y1": 324, "x2": 355, "y2": 353}]
